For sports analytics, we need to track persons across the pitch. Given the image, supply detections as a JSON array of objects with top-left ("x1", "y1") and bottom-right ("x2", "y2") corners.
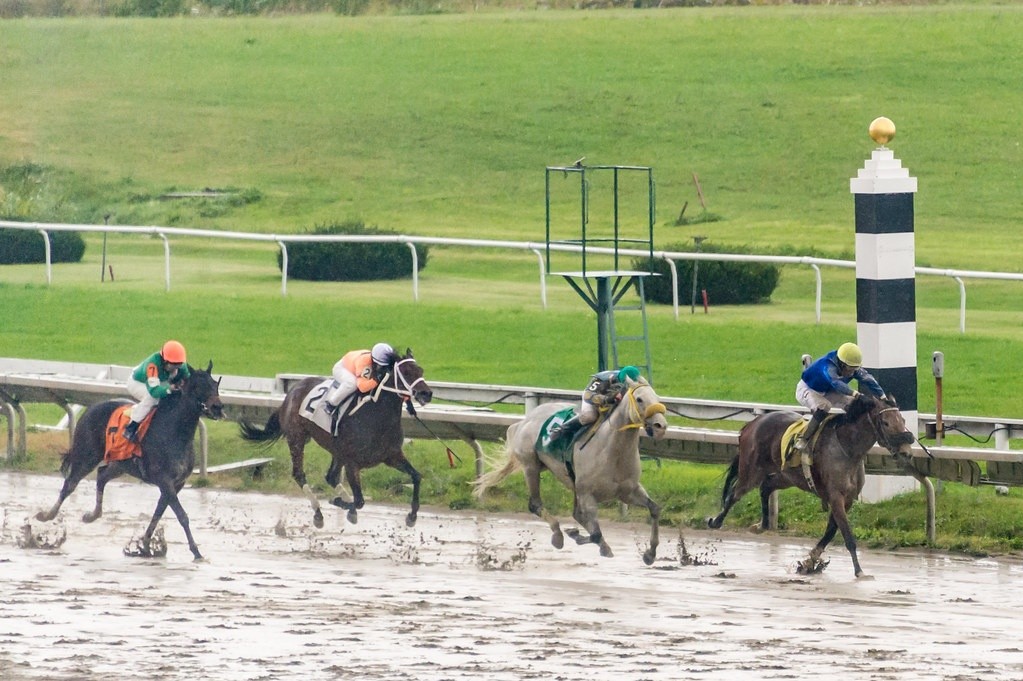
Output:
[
  {"x1": 323, "y1": 343, "x2": 393, "y2": 420},
  {"x1": 550, "y1": 365, "x2": 641, "y2": 444},
  {"x1": 122, "y1": 339, "x2": 191, "y2": 442},
  {"x1": 795, "y1": 342, "x2": 889, "y2": 453}
]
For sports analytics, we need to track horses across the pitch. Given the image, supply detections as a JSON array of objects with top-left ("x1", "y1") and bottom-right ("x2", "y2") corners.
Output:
[
  {"x1": 706, "y1": 390, "x2": 914, "y2": 584},
  {"x1": 36, "y1": 358, "x2": 225, "y2": 563},
  {"x1": 235, "y1": 347, "x2": 431, "y2": 529},
  {"x1": 465, "y1": 374, "x2": 669, "y2": 565}
]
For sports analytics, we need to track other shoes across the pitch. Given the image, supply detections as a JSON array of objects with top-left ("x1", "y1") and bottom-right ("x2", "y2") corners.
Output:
[
  {"x1": 122, "y1": 425, "x2": 139, "y2": 443},
  {"x1": 323, "y1": 399, "x2": 338, "y2": 420}
]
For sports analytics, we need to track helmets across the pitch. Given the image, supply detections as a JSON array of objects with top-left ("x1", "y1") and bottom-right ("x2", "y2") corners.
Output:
[
  {"x1": 618, "y1": 365, "x2": 640, "y2": 384},
  {"x1": 837, "y1": 342, "x2": 862, "y2": 367},
  {"x1": 158, "y1": 340, "x2": 186, "y2": 363},
  {"x1": 371, "y1": 343, "x2": 393, "y2": 366}
]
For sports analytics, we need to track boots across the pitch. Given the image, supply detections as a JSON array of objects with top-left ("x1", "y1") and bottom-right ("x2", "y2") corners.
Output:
[
  {"x1": 549, "y1": 415, "x2": 584, "y2": 444},
  {"x1": 795, "y1": 416, "x2": 819, "y2": 449}
]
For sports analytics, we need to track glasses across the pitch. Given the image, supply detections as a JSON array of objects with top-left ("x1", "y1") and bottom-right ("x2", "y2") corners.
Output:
[{"x1": 845, "y1": 364, "x2": 860, "y2": 371}]
